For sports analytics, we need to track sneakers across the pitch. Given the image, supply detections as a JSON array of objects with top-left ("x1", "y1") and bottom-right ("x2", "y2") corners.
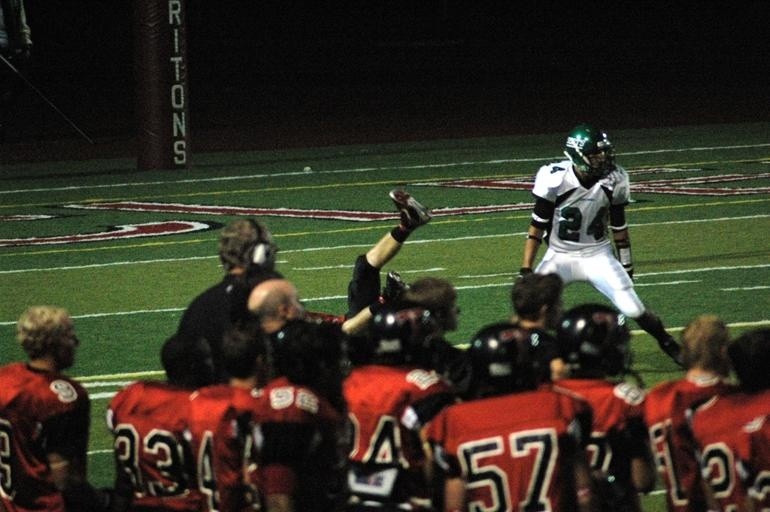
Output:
[{"x1": 388, "y1": 189, "x2": 432, "y2": 231}]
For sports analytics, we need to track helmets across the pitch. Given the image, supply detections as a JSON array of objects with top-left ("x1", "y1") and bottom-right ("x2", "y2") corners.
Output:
[
  {"x1": 552, "y1": 301, "x2": 634, "y2": 380},
  {"x1": 469, "y1": 320, "x2": 539, "y2": 402},
  {"x1": 365, "y1": 307, "x2": 443, "y2": 372},
  {"x1": 269, "y1": 320, "x2": 336, "y2": 388},
  {"x1": 562, "y1": 124, "x2": 616, "y2": 178}
]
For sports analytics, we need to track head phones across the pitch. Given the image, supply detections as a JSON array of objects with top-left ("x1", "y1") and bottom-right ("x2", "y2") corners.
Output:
[{"x1": 244, "y1": 214, "x2": 272, "y2": 267}]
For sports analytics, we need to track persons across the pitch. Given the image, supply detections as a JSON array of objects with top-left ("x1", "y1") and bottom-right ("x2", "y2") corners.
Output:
[
  {"x1": 517, "y1": 124, "x2": 687, "y2": 367},
  {"x1": 104, "y1": 219, "x2": 770, "y2": 511},
  {"x1": 1, "y1": 306, "x2": 111, "y2": 512},
  {"x1": 344, "y1": 187, "x2": 436, "y2": 370}
]
